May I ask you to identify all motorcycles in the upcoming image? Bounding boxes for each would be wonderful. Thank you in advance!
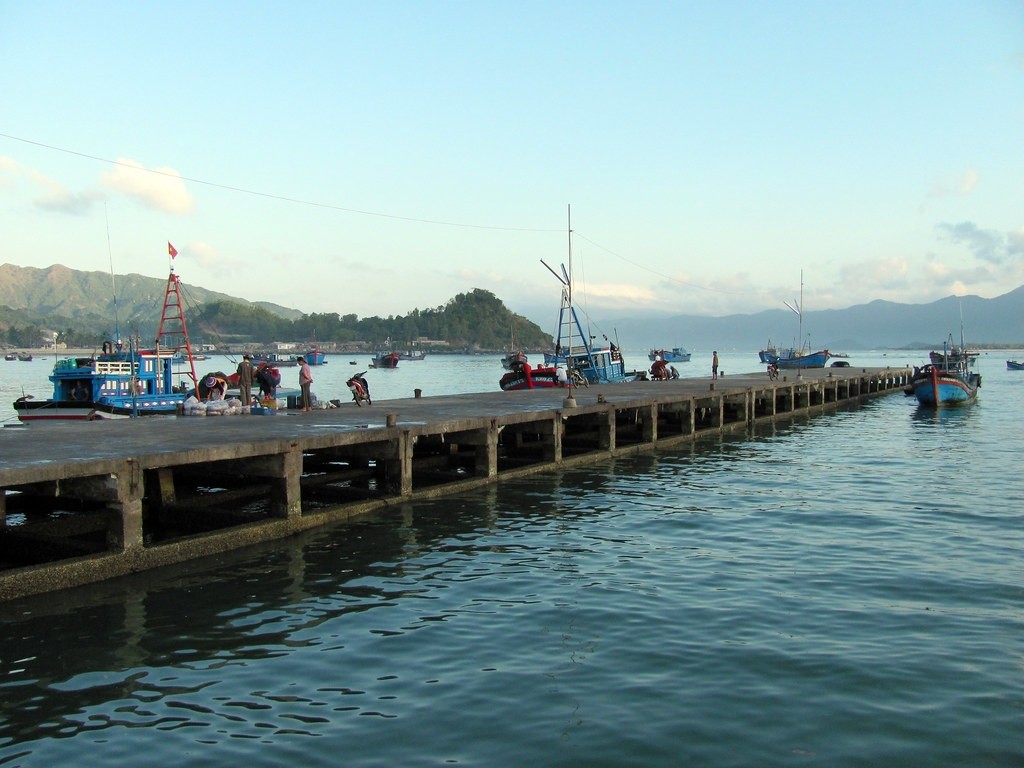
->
[346,371,372,408]
[567,362,590,388]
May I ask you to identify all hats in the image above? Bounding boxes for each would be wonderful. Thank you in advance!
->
[206,377,216,387]
[243,355,249,360]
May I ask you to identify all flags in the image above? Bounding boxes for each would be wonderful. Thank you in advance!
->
[169,243,178,259]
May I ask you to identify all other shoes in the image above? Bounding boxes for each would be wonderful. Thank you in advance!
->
[299,407,311,412]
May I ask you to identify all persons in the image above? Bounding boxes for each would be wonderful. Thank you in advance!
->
[553,367,567,387]
[712,351,718,380]
[296,357,313,412]
[651,351,679,381]
[237,355,254,406]
[253,369,279,397]
[205,377,228,401]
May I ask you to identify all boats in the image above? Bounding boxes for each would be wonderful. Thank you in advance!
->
[396,346,428,362]
[223,387,318,410]
[827,352,851,359]
[563,250,648,386]
[902,300,983,408]
[368,352,401,370]
[542,345,572,363]
[648,344,692,362]
[499,354,568,391]
[242,329,328,367]
[831,361,851,367]
[6,353,32,361]
[13,218,196,424]
[1006,360,1024,370]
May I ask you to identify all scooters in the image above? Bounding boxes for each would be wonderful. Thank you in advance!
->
[767,357,781,380]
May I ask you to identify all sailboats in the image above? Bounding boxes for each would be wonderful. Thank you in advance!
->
[500,324,532,372]
[760,267,830,368]
